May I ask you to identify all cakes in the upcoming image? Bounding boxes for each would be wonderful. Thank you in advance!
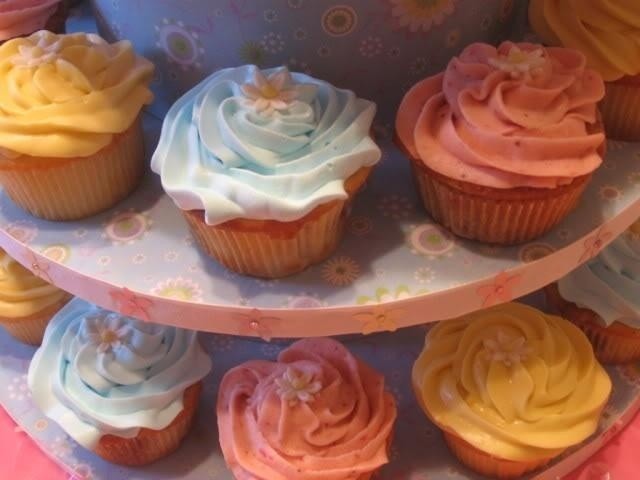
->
[216,336,398,479]
[527,0,640,143]
[149,64,382,278]
[411,300,613,479]
[544,219,640,365]
[0,250,75,347]
[0,1,71,45]
[0,30,156,222]
[28,297,214,466]
[392,39,608,245]
